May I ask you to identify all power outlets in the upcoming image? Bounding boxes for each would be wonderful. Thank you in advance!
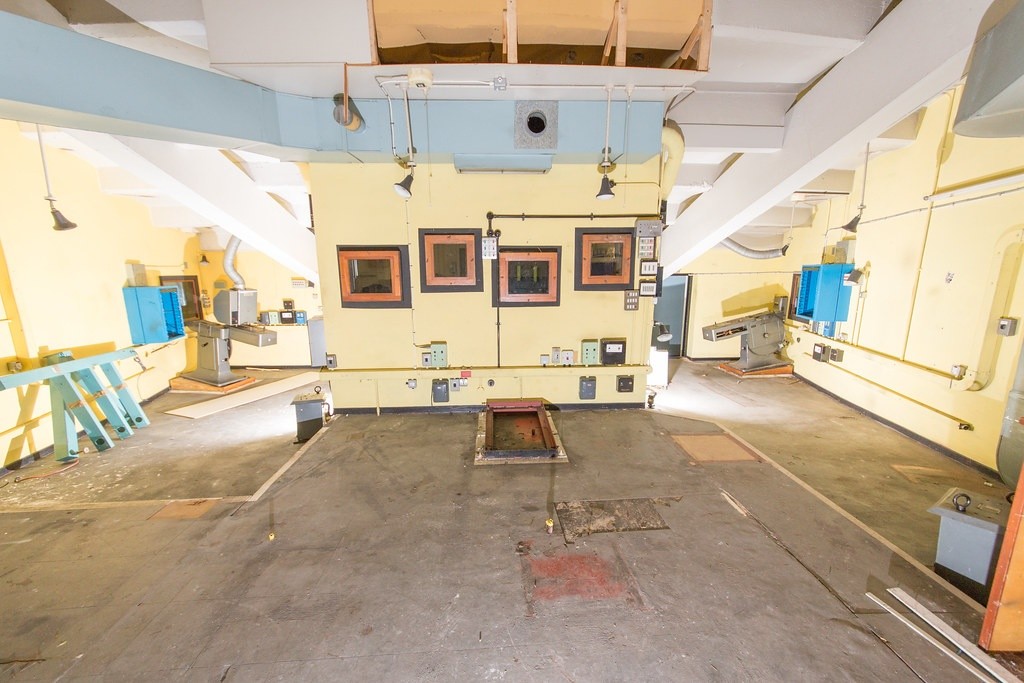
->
[482,237,497,259]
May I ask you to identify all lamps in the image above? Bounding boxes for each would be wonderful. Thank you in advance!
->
[193,253,209,266]
[654,321,673,342]
[597,85,617,199]
[35,123,77,231]
[394,87,416,198]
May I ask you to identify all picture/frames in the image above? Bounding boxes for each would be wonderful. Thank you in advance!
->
[418,228,484,293]
[159,275,204,321]
[574,227,637,292]
[336,244,412,309]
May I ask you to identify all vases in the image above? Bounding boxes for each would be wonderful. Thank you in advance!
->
[491,245,562,307]
